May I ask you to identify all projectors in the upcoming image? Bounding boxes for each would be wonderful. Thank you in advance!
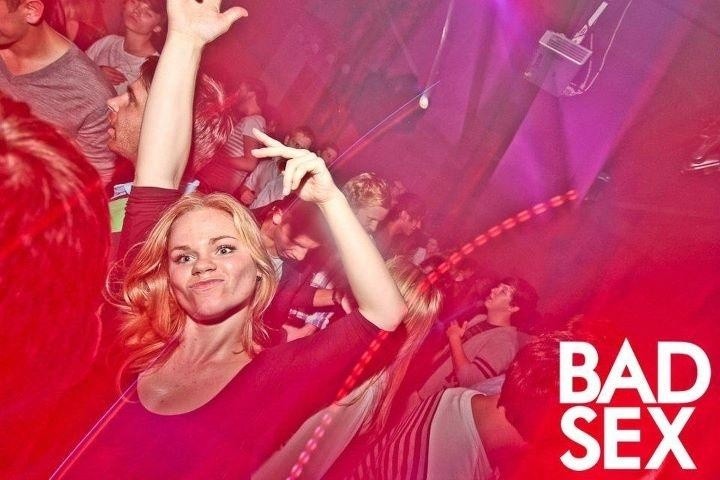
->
[521,29,594,98]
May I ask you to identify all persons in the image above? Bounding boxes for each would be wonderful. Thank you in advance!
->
[2,0,606,479]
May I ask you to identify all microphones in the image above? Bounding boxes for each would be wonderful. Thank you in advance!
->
[585,170,611,202]
